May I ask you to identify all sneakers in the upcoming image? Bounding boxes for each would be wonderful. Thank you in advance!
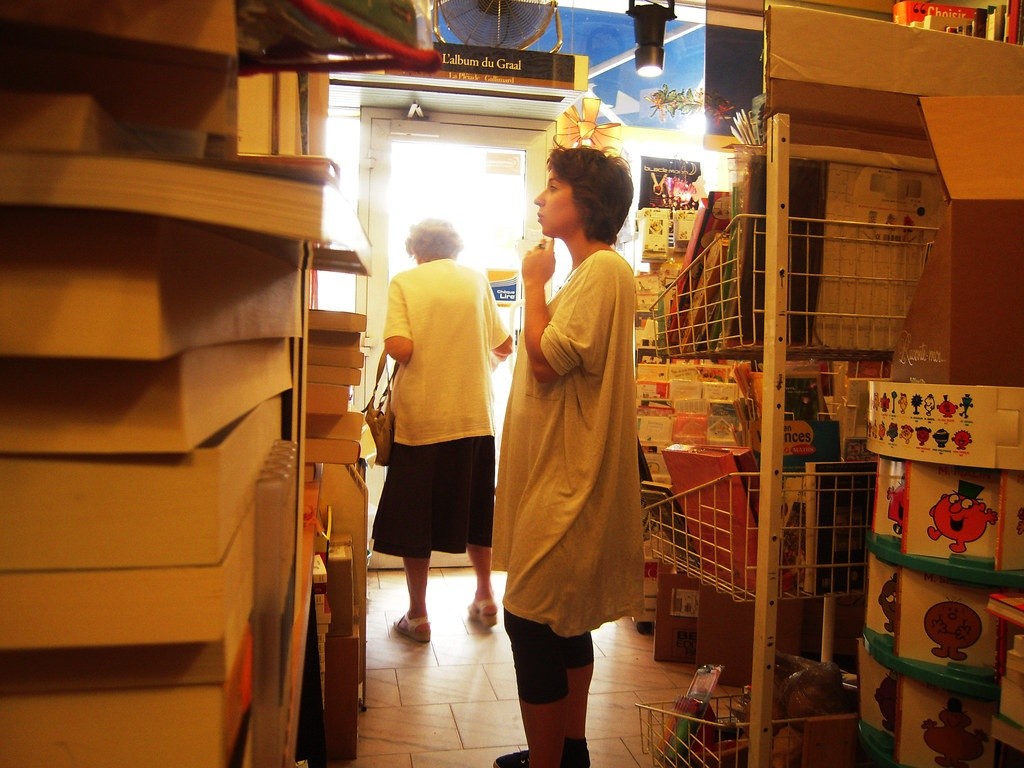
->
[493,750,590,768]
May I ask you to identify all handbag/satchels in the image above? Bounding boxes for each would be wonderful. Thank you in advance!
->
[364,349,401,465]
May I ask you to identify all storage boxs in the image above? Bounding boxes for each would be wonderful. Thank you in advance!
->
[313,532,362,761]
[651,573,803,688]
[1000,635,1024,728]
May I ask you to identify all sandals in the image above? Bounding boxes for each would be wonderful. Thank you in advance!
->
[394,617,431,644]
[469,595,498,626]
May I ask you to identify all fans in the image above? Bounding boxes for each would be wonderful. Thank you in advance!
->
[431,0,564,55]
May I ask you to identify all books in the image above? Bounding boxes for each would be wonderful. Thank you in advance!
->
[633,190,891,594]
[853,452,1024,768]
[0,0,373,768]
[892,0,1024,46]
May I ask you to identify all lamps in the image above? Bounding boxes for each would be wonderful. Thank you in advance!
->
[626,0,678,78]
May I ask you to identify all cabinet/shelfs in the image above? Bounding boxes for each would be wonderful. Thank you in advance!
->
[635,112,945,768]
[856,380,1024,768]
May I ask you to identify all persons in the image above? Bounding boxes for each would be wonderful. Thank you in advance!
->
[373,217,513,643]
[489,134,646,768]
